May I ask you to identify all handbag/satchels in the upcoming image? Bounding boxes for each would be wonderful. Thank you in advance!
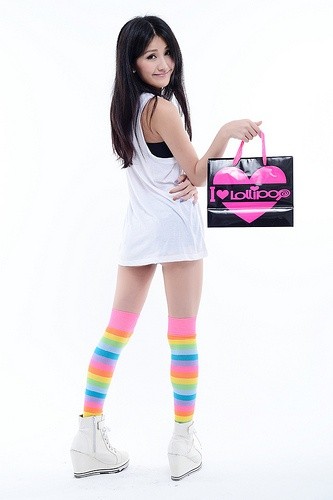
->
[207,130,294,231]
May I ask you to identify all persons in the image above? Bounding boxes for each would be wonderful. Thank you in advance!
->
[72,14,264,479]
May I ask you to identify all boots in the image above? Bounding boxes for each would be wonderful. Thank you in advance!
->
[168,419,203,480]
[68,415,127,478]
